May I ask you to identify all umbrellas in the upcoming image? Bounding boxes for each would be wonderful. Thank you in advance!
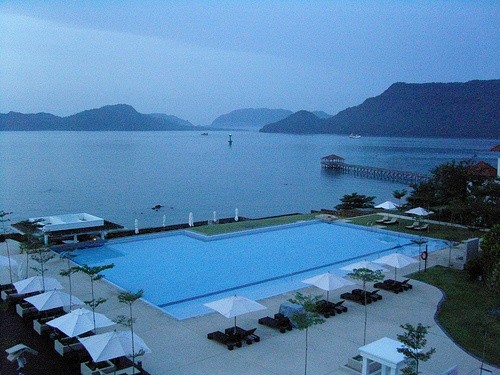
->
[340,258,390,291]
[301,271,354,304]
[12,275,64,294]
[78,329,149,363]
[374,200,402,220]
[45,307,117,338]
[23,287,86,311]
[203,294,267,333]
[371,251,421,282]
[404,206,434,222]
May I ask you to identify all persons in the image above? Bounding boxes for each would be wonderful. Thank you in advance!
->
[16,352,27,375]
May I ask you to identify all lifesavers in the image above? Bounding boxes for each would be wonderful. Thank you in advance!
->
[420,251,428,260]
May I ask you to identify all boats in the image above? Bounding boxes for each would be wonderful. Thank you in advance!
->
[349,134,360,139]
[201,133,208,135]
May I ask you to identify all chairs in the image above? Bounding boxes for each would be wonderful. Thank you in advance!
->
[351,288,383,301]
[224,325,261,346]
[414,223,428,232]
[342,291,372,305]
[405,221,419,230]
[375,216,389,225]
[315,299,347,314]
[311,302,335,318]
[205,330,242,351]
[384,217,398,226]
[257,315,293,332]
[372,282,404,294]
[383,278,413,291]
